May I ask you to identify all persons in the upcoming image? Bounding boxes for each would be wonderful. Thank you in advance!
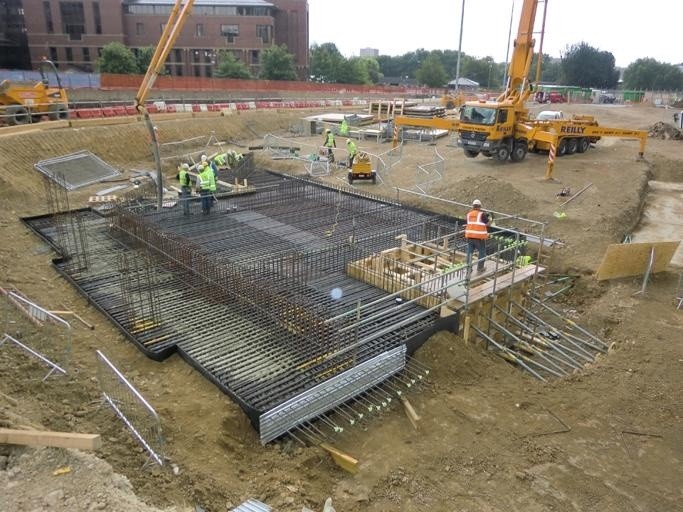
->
[345,139,356,168]
[464,200,492,275]
[174,150,243,214]
[322,129,336,162]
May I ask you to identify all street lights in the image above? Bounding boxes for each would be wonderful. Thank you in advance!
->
[487,60,493,89]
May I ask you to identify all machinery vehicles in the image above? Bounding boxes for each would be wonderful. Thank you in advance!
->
[0,60,69,126]
[386,1,649,182]
[440,90,477,110]
[599,93,616,103]
[534,91,567,104]
[347,152,376,187]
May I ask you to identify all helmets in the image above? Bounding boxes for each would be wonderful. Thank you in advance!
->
[472,199,482,206]
[183,155,208,174]
